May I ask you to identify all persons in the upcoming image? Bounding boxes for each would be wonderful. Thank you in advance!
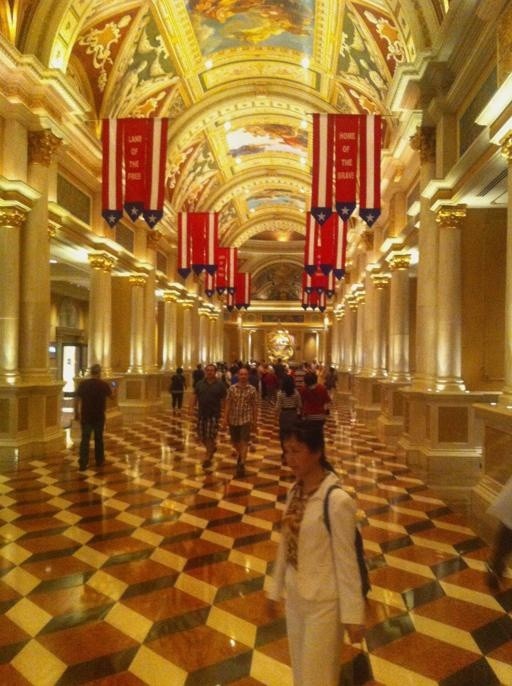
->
[188,356,339,473]
[485,472,512,600]
[268,416,370,684]
[169,367,188,414]
[74,364,118,470]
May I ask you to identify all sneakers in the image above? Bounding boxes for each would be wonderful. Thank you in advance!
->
[202,447,217,467]
[80,460,105,470]
[236,455,245,477]
